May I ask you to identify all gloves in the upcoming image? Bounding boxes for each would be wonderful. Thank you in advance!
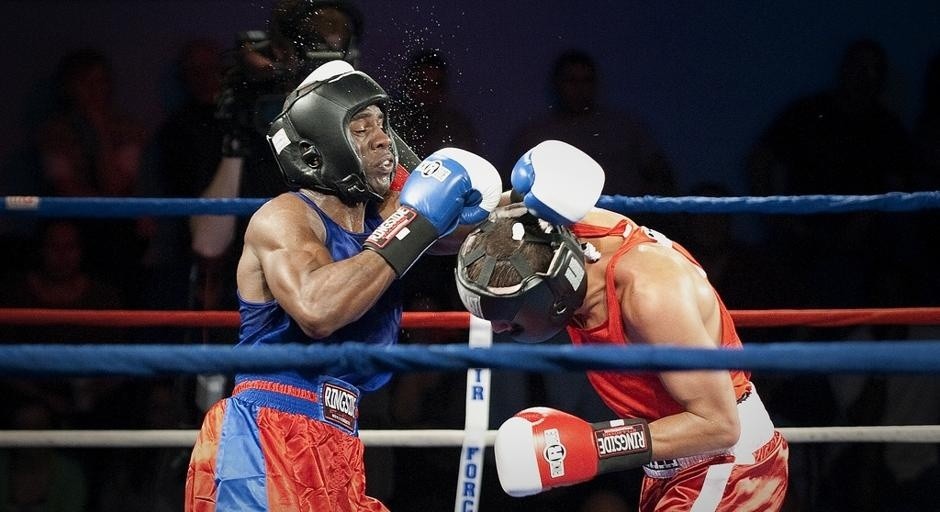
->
[216,71,255,158]
[511,140,606,225]
[396,147,503,237]
[494,405,652,498]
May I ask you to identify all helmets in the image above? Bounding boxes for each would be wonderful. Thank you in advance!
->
[453,215,588,344]
[267,70,398,203]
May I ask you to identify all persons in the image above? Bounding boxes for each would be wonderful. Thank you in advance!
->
[517,35,940,511]
[374,131,788,510]
[360,50,641,510]
[2,1,365,510]
[184,61,605,510]
